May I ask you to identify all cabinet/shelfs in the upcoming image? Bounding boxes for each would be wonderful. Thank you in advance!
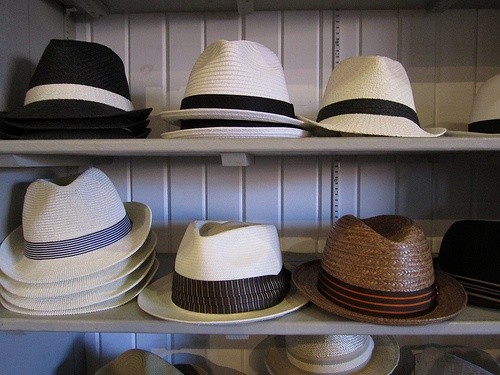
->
[0,138,500,341]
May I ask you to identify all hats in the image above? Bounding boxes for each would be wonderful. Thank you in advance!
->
[0,38,500,375]
